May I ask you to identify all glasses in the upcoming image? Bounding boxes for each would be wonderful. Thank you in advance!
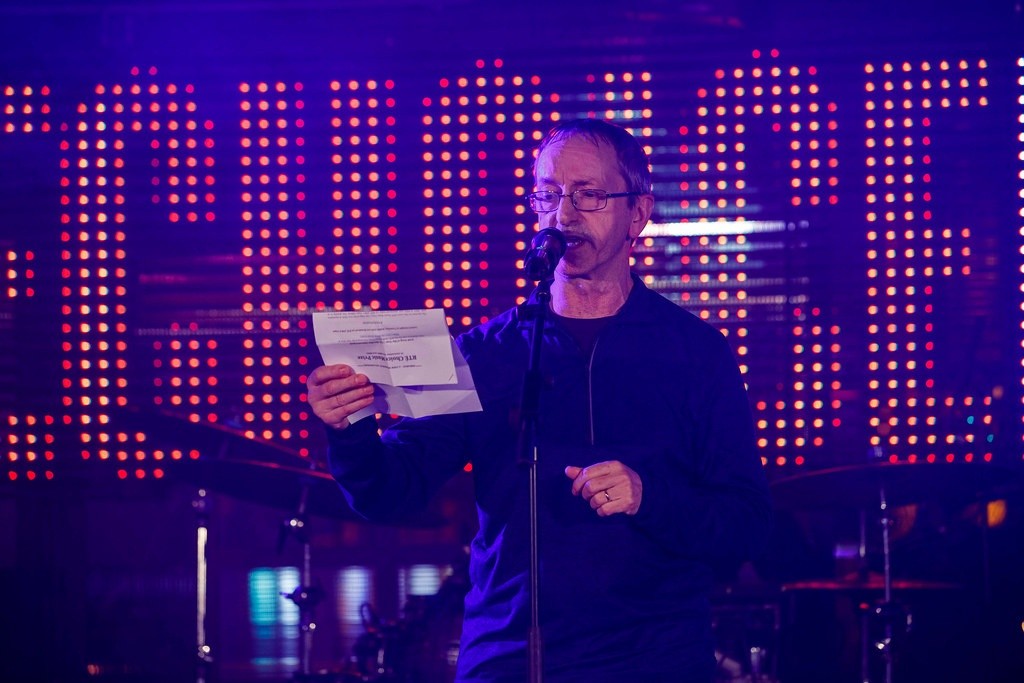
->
[524,186,650,214]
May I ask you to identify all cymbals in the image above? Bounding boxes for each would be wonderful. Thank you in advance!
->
[780,569,960,597]
[179,455,469,532]
[768,455,973,513]
[110,404,314,468]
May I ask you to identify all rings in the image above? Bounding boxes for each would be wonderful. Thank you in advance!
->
[604,490,610,502]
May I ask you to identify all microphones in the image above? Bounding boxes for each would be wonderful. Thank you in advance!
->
[524,225,568,281]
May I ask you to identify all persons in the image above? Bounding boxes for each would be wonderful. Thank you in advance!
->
[306,116,762,682]
